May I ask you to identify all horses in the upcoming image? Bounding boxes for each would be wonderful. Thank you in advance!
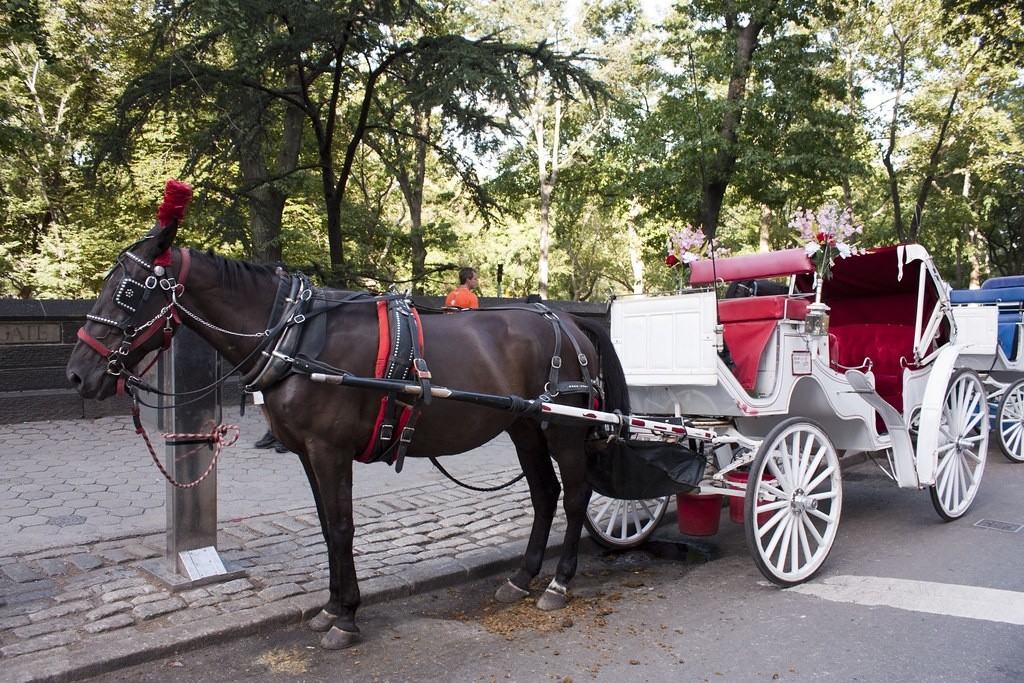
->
[66,224,629,651]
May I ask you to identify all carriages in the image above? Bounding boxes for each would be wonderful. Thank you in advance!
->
[948,275,1023,465]
[65,177,992,650]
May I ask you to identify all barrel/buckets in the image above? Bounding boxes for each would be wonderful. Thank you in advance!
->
[727,472,775,524]
[677,494,722,535]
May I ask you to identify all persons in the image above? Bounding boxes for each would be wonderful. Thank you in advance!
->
[444,265,479,311]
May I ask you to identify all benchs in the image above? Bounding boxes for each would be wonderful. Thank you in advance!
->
[803,291,944,434]
[680,247,817,324]
[951,277,1024,359]
